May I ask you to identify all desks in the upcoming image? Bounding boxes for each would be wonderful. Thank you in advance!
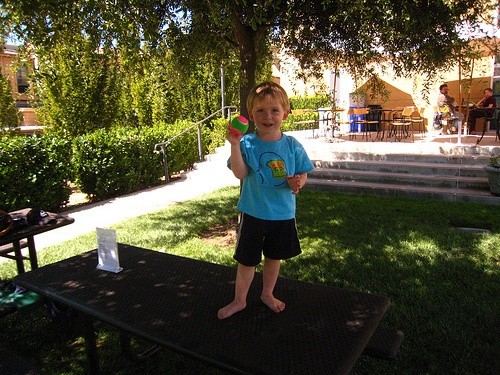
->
[13,241,392,375]
[318,108,403,142]
[454,105,476,134]
[0,206,75,275]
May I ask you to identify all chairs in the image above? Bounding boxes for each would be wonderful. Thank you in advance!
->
[327,106,427,142]
[435,95,500,144]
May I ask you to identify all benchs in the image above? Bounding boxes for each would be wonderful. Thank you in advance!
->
[0,282,48,318]
[362,325,405,362]
[0,240,30,261]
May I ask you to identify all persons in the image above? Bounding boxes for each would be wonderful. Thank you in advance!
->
[437,84,464,131]
[468,88,496,134]
[218,81,315,319]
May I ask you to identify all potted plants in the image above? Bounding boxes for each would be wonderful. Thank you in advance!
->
[484,153,500,195]
